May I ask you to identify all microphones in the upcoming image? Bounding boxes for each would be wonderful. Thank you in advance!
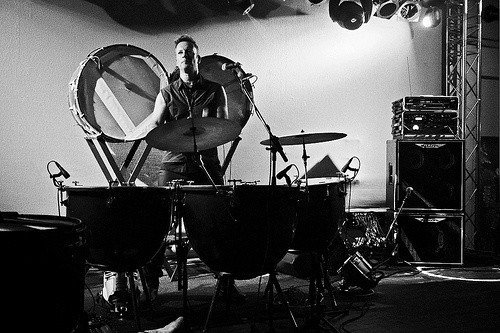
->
[221,62,241,71]
[277,164,293,180]
[341,158,353,173]
[55,162,70,179]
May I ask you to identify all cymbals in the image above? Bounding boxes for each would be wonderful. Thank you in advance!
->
[145,117,242,152]
[260,132,347,145]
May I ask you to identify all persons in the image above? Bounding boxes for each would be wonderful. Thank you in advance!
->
[132,36,249,301]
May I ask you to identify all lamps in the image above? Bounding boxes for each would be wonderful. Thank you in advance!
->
[307,0,441,30]
[226,0,254,15]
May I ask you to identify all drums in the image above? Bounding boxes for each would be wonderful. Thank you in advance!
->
[0,211,86,333]
[181,184,302,280]
[293,179,347,252]
[66,184,175,273]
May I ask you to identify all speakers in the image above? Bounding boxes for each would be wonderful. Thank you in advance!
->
[384,140,466,267]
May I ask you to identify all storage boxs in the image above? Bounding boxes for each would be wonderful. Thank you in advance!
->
[391,95,459,139]
